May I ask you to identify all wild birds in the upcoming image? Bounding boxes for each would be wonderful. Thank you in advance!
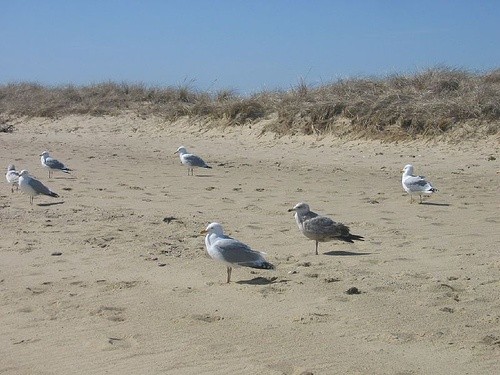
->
[19,170,60,206]
[6,164,21,192]
[40,151,72,178]
[200,222,276,283]
[288,203,365,255]
[174,146,212,176]
[400,165,439,203]
